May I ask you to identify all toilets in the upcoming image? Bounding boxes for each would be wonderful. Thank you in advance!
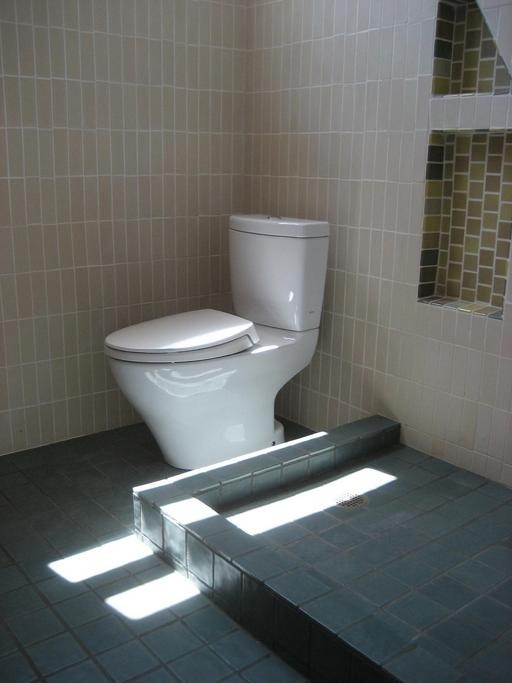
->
[102,212,335,473]
